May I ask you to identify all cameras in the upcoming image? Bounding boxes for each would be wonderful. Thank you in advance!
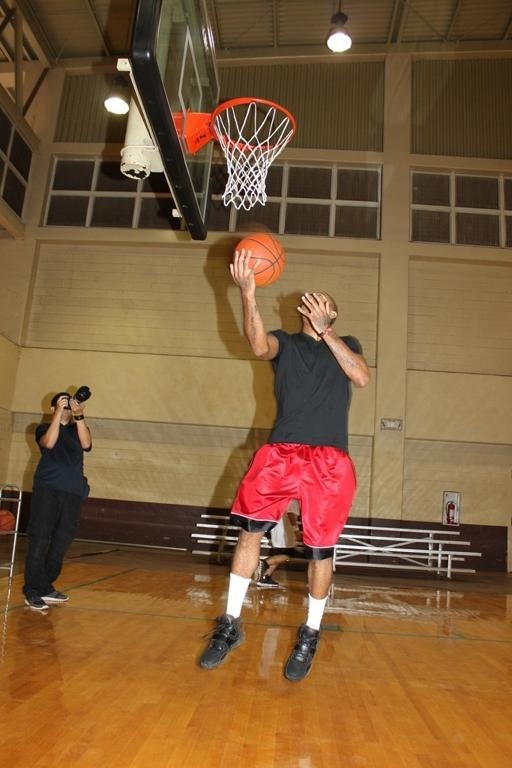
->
[61,386,91,409]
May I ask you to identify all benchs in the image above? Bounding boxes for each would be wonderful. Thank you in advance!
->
[188,511,481,581]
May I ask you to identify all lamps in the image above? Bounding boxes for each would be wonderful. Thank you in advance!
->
[325,0,353,54]
[100,78,130,118]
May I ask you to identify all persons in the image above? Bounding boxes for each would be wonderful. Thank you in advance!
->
[21,392,92,609]
[199,247,370,682]
[253,496,300,587]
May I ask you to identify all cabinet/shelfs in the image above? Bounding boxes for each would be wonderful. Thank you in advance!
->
[0,482,22,578]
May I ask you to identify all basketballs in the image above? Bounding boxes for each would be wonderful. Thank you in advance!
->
[0,510,15,531]
[233,233,286,288]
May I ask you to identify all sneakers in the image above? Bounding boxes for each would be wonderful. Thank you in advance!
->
[200,613,245,668]
[41,591,70,602]
[284,623,321,681]
[257,575,278,587]
[23,596,48,610]
[255,559,269,581]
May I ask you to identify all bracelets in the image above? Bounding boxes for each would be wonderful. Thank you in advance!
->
[293,525,299,531]
[74,415,84,420]
[319,326,332,337]
